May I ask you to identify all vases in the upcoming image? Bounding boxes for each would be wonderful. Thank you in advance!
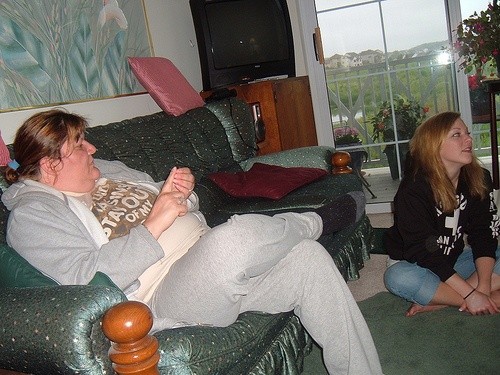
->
[491,54,500,78]
[385,148,410,181]
[336,139,363,174]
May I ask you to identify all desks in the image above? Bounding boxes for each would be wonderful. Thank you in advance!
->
[481,78,500,191]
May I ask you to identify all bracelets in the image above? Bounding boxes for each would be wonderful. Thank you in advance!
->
[464,288,475,300]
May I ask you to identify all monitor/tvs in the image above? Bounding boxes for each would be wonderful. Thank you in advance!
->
[189,0,295,91]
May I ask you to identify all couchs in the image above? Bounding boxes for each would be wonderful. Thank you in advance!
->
[0,92,376,375]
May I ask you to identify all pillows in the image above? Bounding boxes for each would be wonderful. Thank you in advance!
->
[128,57,206,117]
[206,162,328,199]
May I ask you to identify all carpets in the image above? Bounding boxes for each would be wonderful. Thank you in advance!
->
[301,292,500,375]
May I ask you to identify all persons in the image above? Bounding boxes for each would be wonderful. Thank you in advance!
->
[0,108,385,375]
[384,111,500,316]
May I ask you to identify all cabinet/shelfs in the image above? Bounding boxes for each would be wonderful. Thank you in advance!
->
[198,75,317,156]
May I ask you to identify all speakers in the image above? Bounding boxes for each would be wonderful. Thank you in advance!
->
[247,102,265,143]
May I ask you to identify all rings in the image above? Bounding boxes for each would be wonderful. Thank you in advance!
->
[189,187,194,191]
[178,199,181,204]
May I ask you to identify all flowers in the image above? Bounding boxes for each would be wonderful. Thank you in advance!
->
[363,94,430,151]
[447,0,500,91]
[333,121,361,144]
